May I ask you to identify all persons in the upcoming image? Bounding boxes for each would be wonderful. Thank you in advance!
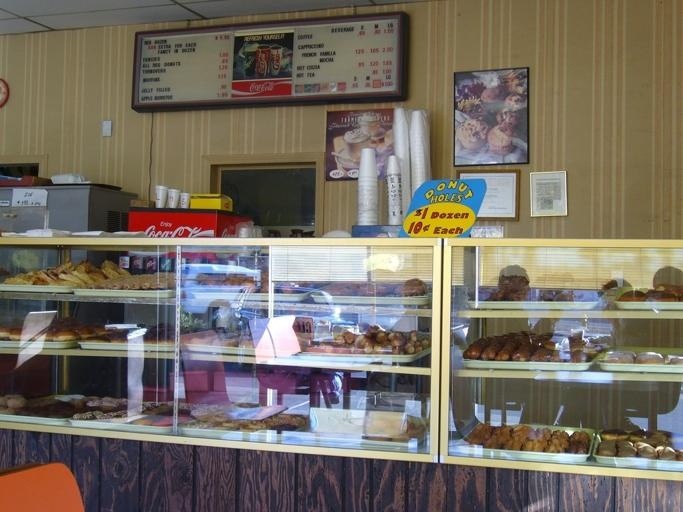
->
[364,262,683,432]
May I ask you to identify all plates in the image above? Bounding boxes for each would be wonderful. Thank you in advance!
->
[335,149,377,169]
[355,127,386,141]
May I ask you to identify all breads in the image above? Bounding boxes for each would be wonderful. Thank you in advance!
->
[457,86,527,154]
[463,331,683,366]
[180,405,429,441]
[2,316,175,343]
[466,423,683,461]
[4,261,186,291]
[196,273,426,297]
[469,285,683,302]
[213,321,431,355]
[2,393,194,425]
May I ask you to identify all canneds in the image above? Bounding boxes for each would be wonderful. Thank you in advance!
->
[269,47,281,76]
[256,45,269,76]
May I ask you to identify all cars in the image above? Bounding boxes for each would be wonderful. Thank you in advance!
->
[170,264,342,340]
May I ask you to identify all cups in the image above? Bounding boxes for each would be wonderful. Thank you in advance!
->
[392,106,433,210]
[357,148,378,226]
[357,116,380,134]
[154,186,190,209]
[385,154,404,226]
[344,130,371,159]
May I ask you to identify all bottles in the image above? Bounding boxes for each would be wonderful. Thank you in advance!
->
[244,40,260,76]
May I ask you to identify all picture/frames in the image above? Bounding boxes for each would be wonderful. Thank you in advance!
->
[130,11,408,113]
[454,169,567,222]
[451,66,529,166]
